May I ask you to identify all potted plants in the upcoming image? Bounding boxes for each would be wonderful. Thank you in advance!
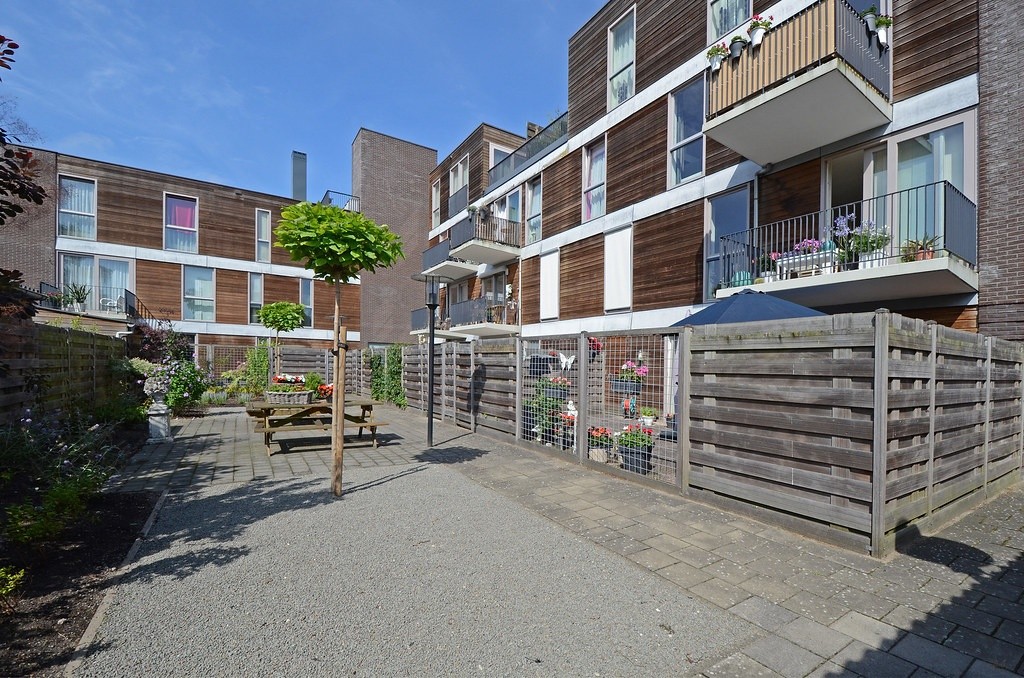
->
[861,6,892,46]
[62,284,92,311]
[464,205,492,220]
[902,231,943,260]
[642,407,657,427]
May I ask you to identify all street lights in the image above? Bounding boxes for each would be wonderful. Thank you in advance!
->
[411,272,461,447]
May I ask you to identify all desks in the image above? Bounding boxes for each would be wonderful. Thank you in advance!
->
[246,402,384,455]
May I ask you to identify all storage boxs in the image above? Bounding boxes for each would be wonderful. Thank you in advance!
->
[266,381,315,404]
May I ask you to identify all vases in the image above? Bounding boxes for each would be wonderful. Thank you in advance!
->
[51,301,60,310]
[756,250,887,286]
[538,381,652,475]
[695,28,772,71]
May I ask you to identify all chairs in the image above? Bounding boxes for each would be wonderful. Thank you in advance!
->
[100,295,124,314]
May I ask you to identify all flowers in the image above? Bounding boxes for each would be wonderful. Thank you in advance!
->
[46,291,61,300]
[262,371,337,401]
[709,13,772,52]
[759,213,886,261]
[544,340,659,448]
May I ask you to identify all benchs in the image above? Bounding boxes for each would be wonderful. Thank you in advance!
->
[250,409,391,459]
